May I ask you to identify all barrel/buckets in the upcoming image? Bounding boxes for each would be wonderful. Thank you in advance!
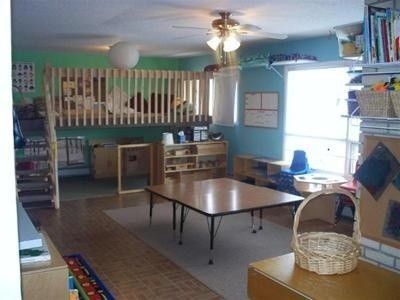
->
[161,132,174,145]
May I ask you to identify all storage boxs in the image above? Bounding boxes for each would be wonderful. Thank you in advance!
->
[333,20,362,61]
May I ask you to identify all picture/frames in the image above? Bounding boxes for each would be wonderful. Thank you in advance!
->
[12,61,35,93]
[244,90,279,129]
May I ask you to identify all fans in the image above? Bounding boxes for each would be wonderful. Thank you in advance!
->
[171,10,289,40]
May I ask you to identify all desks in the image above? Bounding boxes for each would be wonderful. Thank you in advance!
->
[294,172,349,224]
[247,252,400,300]
[145,177,305,266]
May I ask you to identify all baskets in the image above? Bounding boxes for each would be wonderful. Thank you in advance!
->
[290,188,361,275]
[355,90,400,118]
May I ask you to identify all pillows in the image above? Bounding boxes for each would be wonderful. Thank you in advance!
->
[124,92,174,113]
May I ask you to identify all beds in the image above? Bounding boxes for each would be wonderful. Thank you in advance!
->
[53,95,212,125]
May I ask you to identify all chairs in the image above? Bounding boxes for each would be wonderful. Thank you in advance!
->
[278,150,311,192]
[333,160,360,226]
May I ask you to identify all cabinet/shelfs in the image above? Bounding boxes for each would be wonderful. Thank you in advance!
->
[151,140,229,206]
[355,1,400,136]
[90,148,152,181]
[233,154,290,210]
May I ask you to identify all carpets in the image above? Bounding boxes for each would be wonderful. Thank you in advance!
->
[102,202,301,300]
[64,253,113,300]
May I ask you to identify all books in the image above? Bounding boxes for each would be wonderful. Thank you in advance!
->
[364,5,400,65]
